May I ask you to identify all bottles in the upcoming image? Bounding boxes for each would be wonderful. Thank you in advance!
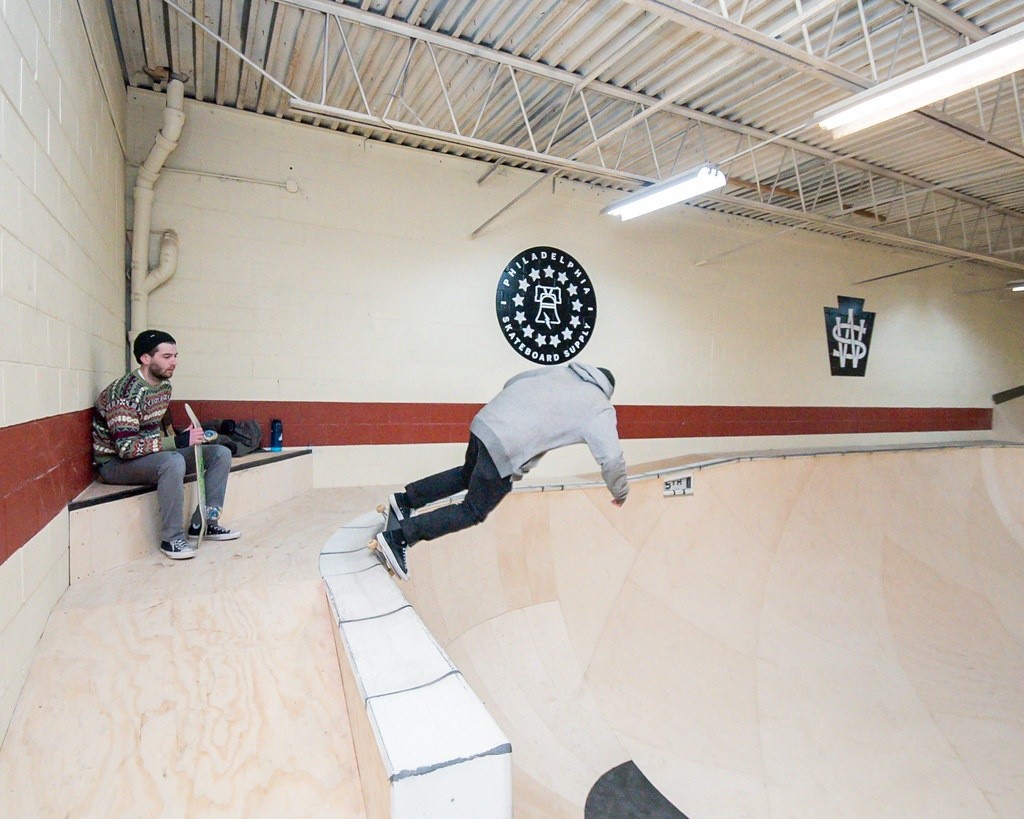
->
[270,418,282,452]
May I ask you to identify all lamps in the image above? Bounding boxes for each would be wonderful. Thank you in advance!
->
[1006,279,1024,291]
[812,21,1024,141]
[599,162,726,222]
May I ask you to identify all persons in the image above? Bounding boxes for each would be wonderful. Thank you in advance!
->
[93,330,240,559]
[376,362,631,581]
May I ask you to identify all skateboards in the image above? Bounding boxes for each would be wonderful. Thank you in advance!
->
[367,502,410,581]
[184,402,222,551]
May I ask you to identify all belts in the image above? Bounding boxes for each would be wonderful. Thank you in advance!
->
[99,460,111,468]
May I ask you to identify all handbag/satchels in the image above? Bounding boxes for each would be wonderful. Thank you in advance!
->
[201,419,261,457]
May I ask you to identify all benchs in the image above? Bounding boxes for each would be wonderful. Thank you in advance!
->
[69,449,313,584]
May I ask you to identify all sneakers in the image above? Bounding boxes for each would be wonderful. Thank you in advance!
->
[376,529,409,579]
[188,522,241,541]
[159,538,196,559]
[390,492,412,522]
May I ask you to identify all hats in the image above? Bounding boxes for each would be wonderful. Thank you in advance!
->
[133,330,176,364]
[598,367,615,388]
